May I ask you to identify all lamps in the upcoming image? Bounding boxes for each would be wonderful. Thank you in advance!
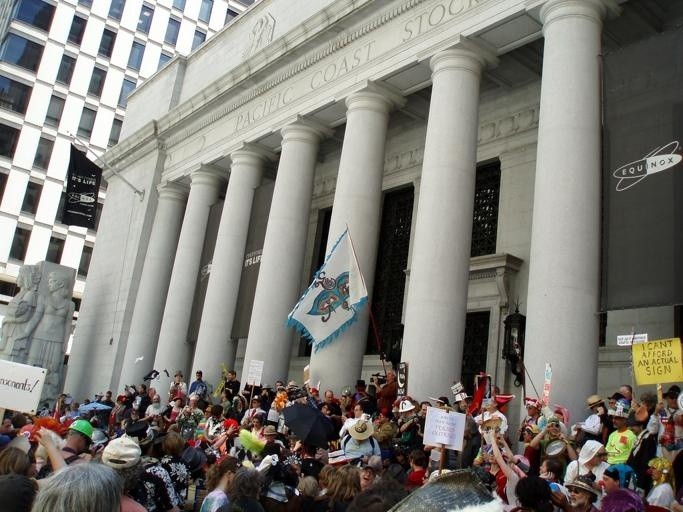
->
[502,296,526,388]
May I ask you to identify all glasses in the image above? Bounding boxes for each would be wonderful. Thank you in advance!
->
[595,453,607,457]
[568,488,580,493]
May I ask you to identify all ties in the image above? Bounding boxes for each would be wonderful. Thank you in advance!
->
[251,409,256,417]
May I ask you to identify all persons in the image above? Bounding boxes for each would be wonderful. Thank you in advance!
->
[13,271,76,385]
[0,263,40,365]
[1,368,683,510]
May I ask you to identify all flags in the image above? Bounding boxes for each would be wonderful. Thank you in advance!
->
[62,143,103,229]
[286,229,369,354]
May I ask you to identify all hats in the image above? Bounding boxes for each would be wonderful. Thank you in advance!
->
[663,385,680,398]
[8,420,158,469]
[252,395,262,400]
[182,448,206,472]
[453,393,473,404]
[260,384,273,389]
[587,392,630,418]
[263,425,277,434]
[565,470,598,496]
[355,380,366,387]
[224,419,238,430]
[525,398,542,410]
[398,400,415,412]
[363,465,377,476]
[349,420,374,440]
[604,463,637,490]
